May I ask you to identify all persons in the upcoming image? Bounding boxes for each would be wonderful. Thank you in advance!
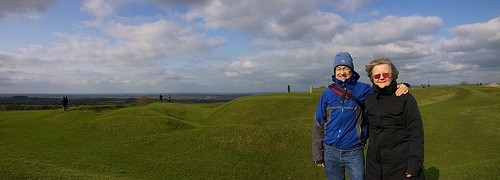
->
[160,94,163,102]
[312,52,412,180]
[62,96,69,112]
[362,58,427,180]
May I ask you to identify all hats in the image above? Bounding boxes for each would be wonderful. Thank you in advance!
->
[333,52,354,72]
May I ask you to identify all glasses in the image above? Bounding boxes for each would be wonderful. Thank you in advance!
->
[371,73,393,79]
